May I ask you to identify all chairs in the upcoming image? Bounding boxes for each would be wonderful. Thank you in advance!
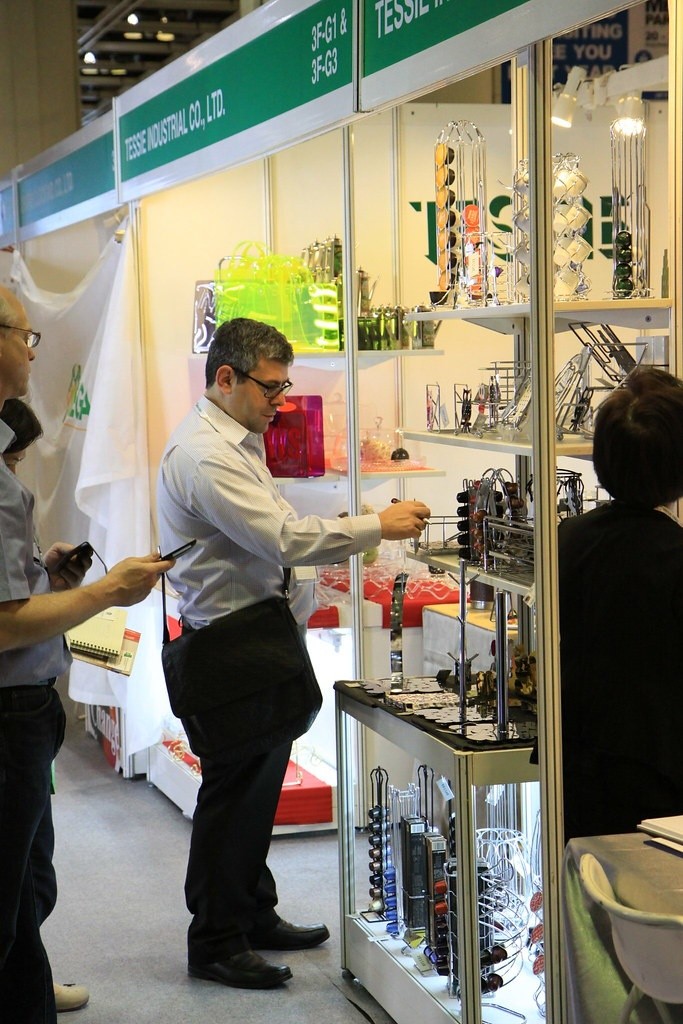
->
[580,853,683,1024]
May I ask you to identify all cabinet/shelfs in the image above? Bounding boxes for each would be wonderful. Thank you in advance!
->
[402,300,672,677]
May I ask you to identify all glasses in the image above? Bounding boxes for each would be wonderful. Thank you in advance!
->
[230,364,293,398]
[0,324,41,348]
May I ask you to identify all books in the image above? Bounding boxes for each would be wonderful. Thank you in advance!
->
[62,607,141,676]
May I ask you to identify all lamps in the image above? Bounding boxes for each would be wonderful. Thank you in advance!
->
[610,87,645,135]
[551,67,585,127]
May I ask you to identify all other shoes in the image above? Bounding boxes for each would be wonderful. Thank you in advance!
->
[52,981,88,1010]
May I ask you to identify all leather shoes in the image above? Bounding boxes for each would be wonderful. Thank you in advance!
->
[187,950,292,989]
[249,913,330,950]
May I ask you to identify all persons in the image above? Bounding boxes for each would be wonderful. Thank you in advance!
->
[0,281,176,1024]
[157,315,431,989]
[529,369,682,844]
[1,397,89,1011]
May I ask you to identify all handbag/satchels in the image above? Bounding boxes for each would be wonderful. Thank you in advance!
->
[161,596,324,762]
